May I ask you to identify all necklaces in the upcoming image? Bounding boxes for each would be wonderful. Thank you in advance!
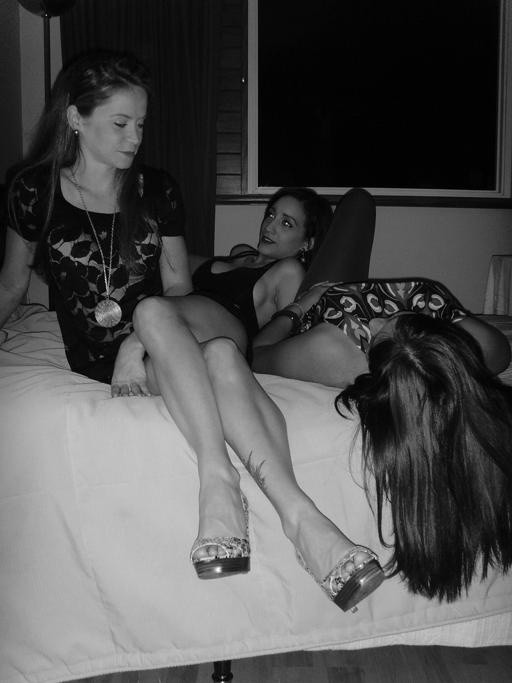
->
[61,169,124,326]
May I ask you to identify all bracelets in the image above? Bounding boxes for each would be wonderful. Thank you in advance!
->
[284,302,304,319]
[272,309,302,334]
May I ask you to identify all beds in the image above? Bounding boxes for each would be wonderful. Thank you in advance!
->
[1,295,511,680]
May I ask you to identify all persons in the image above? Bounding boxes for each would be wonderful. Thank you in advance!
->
[108,182,332,398]
[0,63,385,614]
[244,276,512,598]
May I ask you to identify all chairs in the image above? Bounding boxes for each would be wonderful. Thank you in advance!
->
[294,188,376,309]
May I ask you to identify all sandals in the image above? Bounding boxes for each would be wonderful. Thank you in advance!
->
[191,490,252,579]
[294,542,387,612]
